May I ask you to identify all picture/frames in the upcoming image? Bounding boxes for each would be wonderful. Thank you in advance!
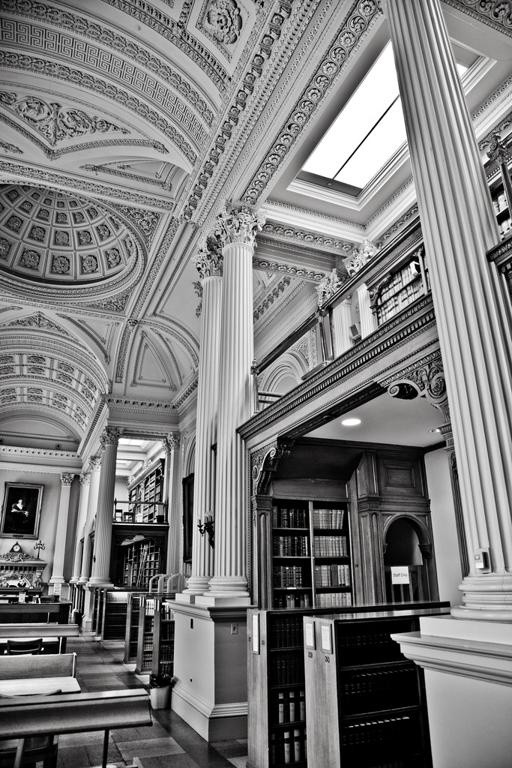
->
[0,481,45,541]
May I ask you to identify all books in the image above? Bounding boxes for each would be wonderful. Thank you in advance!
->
[269,500,356,688]
[127,469,164,525]
[105,599,174,679]
[123,553,159,587]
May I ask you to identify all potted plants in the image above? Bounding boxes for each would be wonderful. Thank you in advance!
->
[146,674,174,711]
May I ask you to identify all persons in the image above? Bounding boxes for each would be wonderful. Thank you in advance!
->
[13,498,28,511]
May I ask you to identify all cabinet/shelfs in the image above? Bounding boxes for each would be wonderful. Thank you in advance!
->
[104,440,173,682]
[253,474,349,611]
[243,608,380,767]
[302,607,453,767]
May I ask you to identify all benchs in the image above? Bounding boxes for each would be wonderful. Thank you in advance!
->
[0,587,153,768]
